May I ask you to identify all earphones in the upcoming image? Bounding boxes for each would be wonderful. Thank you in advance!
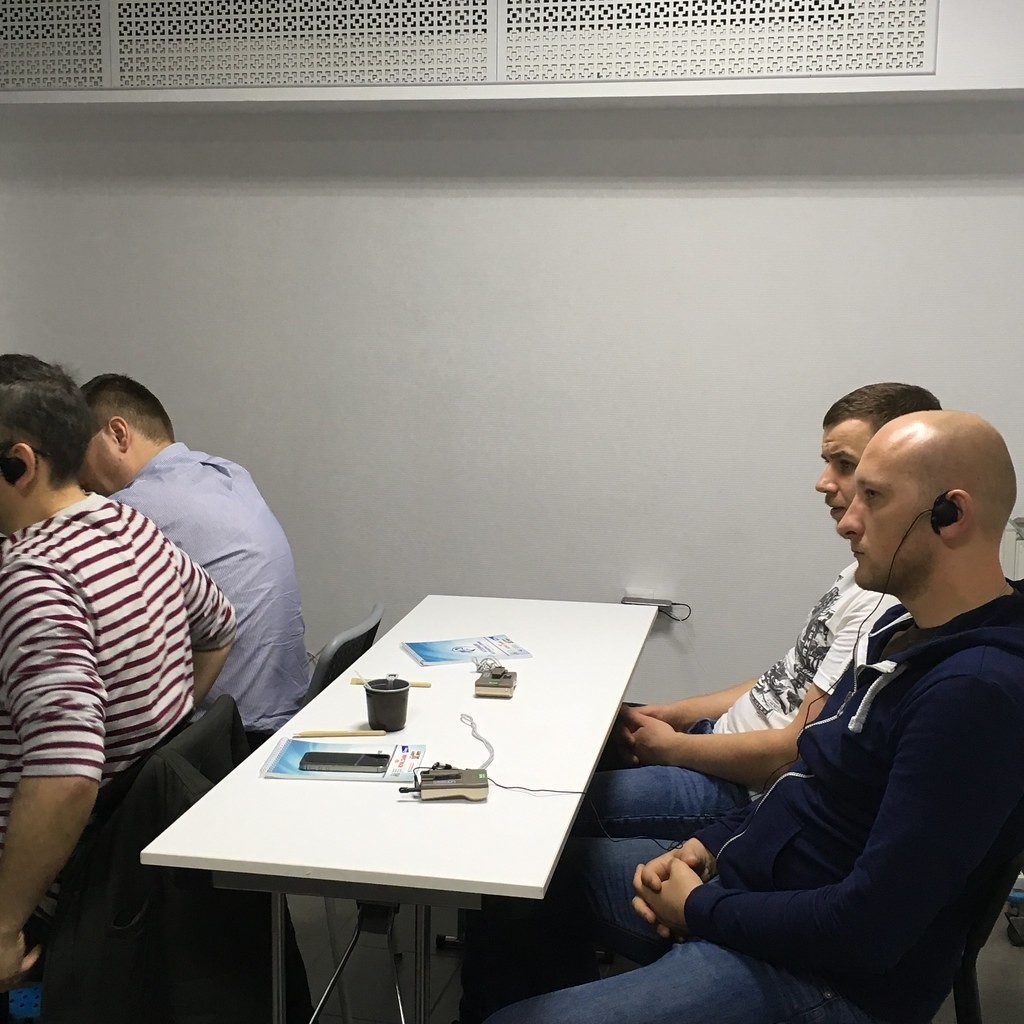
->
[0,456,26,484]
[931,490,960,535]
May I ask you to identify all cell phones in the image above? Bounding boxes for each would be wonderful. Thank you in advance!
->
[300,752,391,772]
[621,597,672,607]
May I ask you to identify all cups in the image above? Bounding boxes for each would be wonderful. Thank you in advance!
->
[365,679,410,731]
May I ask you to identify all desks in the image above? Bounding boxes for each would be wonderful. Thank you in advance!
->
[141,589,660,1024]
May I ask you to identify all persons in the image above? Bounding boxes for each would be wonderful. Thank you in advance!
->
[458,383,1024,1024]
[77,372,311,755]
[0,354,239,993]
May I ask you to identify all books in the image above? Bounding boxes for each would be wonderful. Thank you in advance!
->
[259,738,426,782]
[401,634,532,666]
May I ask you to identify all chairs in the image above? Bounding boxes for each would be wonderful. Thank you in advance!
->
[0,598,386,1024]
[951,797,1023,1023]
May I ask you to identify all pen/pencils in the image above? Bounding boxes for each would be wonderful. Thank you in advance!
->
[351,677,432,687]
[292,730,386,736]
